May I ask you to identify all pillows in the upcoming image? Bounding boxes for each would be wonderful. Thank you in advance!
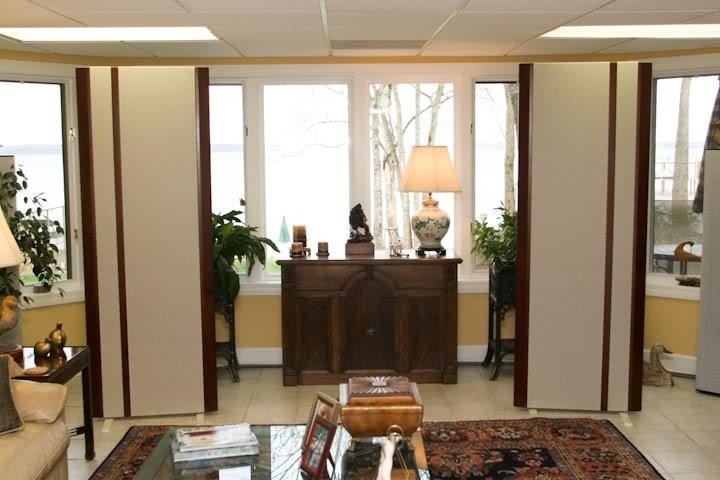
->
[0,354,24,432]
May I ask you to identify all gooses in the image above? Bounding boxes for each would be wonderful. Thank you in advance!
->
[642,342,674,387]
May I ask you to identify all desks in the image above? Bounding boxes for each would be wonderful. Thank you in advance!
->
[652,243,702,275]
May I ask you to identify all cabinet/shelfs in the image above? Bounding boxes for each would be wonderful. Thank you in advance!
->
[276,258,462,389]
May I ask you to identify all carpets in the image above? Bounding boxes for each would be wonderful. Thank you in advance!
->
[85,418,664,480]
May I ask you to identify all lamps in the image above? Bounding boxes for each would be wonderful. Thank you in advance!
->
[396,144,464,257]
[0,202,31,434]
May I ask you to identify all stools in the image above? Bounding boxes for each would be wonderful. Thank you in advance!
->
[334,373,431,479]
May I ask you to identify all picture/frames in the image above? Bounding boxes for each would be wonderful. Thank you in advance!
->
[301,418,337,480]
[301,391,341,452]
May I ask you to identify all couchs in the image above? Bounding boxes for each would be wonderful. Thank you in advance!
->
[0,379,73,480]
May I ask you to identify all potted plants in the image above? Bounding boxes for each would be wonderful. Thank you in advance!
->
[209,211,280,382]
[472,202,518,382]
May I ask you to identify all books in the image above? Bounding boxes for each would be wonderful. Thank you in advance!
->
[169,421,261,462]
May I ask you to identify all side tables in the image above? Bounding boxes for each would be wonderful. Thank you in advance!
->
[11,344,97,464]
